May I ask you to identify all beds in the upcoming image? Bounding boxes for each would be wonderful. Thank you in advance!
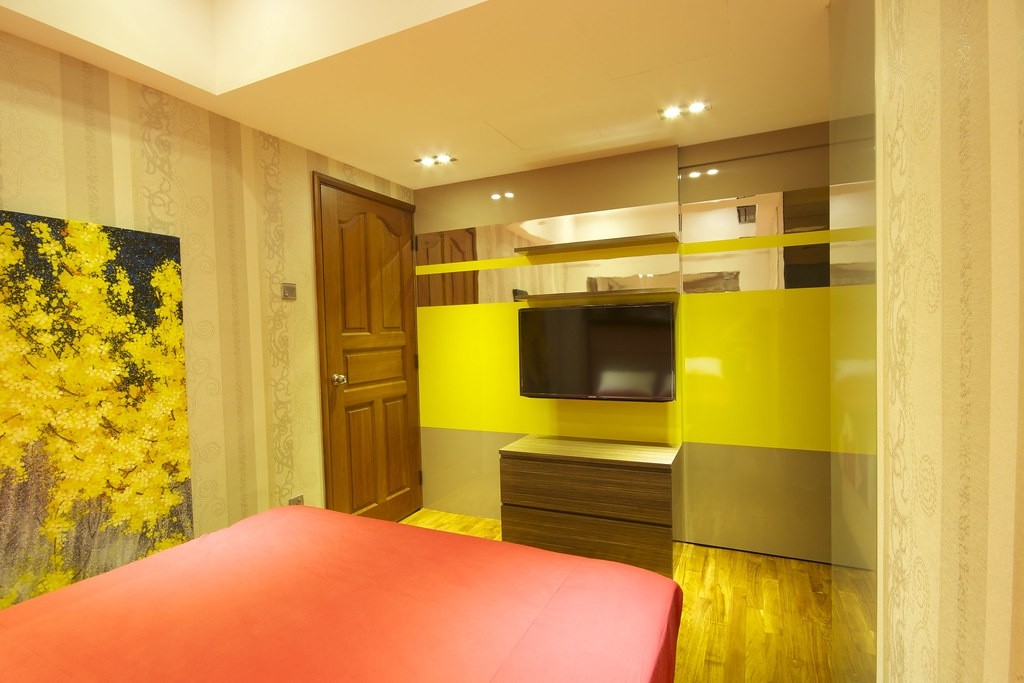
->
[0,505,683,683]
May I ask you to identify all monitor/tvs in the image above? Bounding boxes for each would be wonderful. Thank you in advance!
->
[518,301,675,402]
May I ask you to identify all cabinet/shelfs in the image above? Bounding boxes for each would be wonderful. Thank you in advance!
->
[499,433,684,580]
